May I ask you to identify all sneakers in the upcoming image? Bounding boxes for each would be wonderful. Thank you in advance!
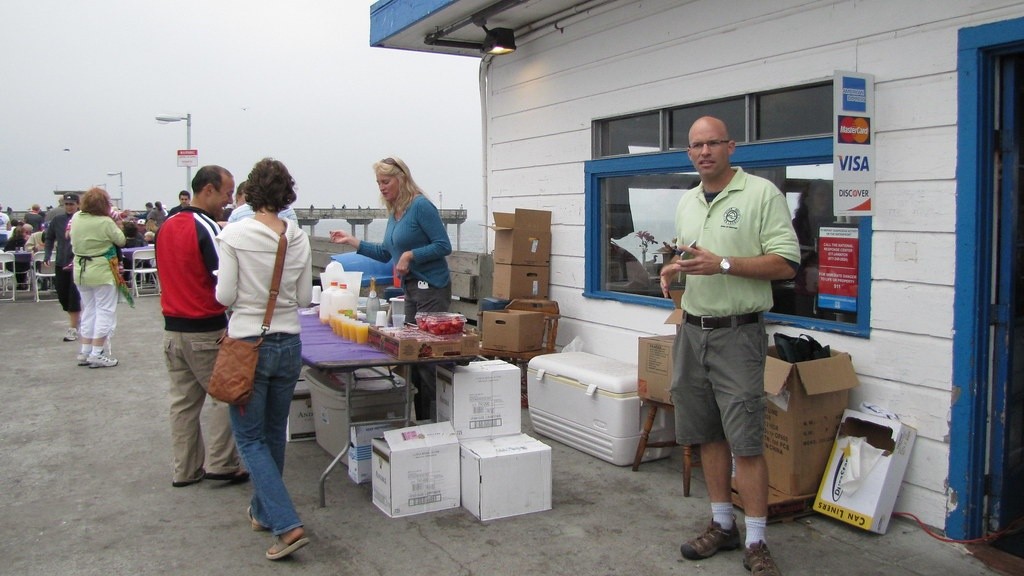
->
[680,519,742,560]
[744,540,782,575]
[87,350,118,368]
[63,328,79,341]
[77,351,92,366]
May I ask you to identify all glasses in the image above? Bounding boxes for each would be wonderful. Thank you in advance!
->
[381,158,406,176]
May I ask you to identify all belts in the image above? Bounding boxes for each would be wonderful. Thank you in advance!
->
[681,311,764,330]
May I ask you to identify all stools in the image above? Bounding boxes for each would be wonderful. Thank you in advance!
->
[633,398,709,497]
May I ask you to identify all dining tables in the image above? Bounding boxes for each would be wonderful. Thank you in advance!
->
[291,311,485,509]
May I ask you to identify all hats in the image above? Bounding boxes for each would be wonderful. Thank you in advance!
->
[63,192,79,204]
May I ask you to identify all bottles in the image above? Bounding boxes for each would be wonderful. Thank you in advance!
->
[15,247,23,254]
[366,277,381,324]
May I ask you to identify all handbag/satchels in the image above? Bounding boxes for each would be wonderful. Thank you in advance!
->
[206,331,263,406]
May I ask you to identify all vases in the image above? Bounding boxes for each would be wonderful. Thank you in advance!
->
[641,249,650,274]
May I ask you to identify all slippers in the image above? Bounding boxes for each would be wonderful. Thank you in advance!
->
[205,467,251,481]
[248,505,268,530]
[172,470,205,486]
[265,536,311,559]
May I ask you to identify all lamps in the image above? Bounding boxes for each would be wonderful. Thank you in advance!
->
[474,16,517,57]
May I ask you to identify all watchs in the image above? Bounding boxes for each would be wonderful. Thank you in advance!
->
[719,255,731,275]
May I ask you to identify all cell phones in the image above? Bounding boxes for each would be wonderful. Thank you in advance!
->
[679,240,696,282]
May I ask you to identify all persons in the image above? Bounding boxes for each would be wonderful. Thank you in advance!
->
[215,178,251,228]
[74,186,127,369]
[215,157,316,561]
[1,176,193,296]
[657,113,806,576]
[227,203,300,231]
[329,155,452,422]
[43,192,81,342]
[152,164,252,490]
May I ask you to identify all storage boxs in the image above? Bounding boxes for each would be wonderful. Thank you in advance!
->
[434,359,521,440]
[721,475,806,519]
[479,307,546,351]
[636,333,699,406]
[482,345,549,407]
[284,368,318,443]
[524,350,679,466]
[452,433,557,522]
[490,251,553,304]
[755,342,859,496]
[345,421,418,487]
[368,324,485,362]
[478,207,554,265]
[303,363,419,463]
[369,418,462,519]
[501,297,562,351]
[664,288,693,335]
[811,408,917,535]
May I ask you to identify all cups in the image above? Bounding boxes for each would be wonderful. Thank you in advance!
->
[328,312,370,344]
[376,311,387,326]
[311,286,322,304]
[392,314,406,327]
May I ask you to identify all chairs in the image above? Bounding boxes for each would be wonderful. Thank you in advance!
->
[0,243,171,303]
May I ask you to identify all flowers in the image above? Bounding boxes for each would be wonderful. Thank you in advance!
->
[631,230,659,253]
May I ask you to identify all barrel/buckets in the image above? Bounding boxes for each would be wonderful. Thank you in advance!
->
[319,282,357,324]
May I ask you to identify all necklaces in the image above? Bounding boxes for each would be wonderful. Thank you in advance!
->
[256,211,267,217]
